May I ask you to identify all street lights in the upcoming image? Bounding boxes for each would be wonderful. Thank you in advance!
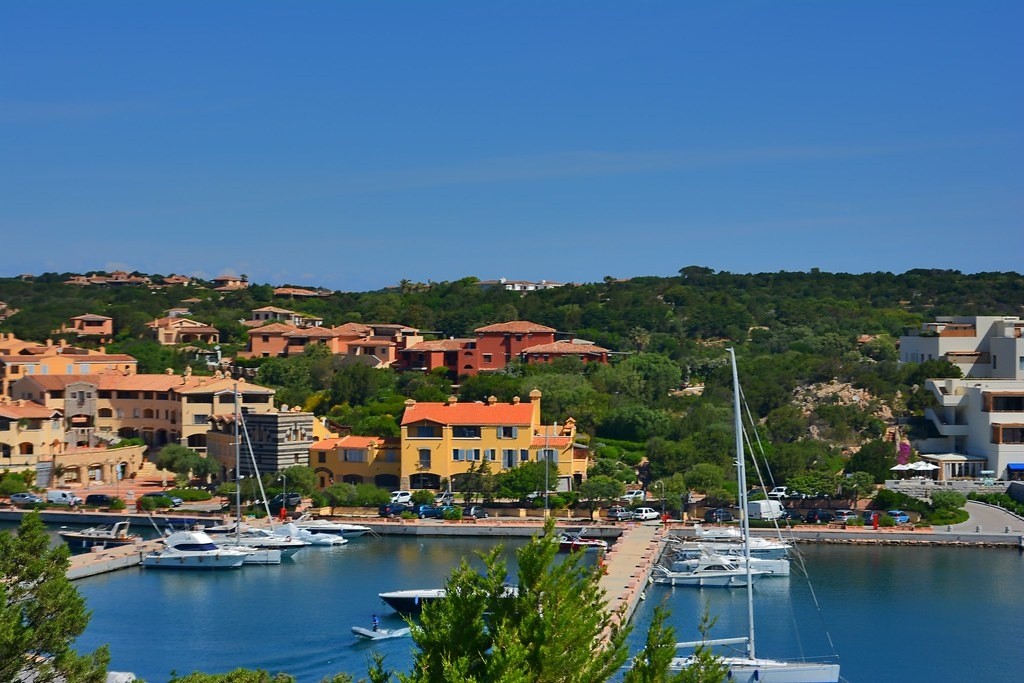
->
[277,474,286,508]
[654,481,665,515]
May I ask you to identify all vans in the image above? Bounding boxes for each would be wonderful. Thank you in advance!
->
[47,490,82,504]
[747,501,785,521]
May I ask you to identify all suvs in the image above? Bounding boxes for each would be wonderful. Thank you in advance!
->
[86,494,116,506]
[143,492,183,508]
[607,508,660,522]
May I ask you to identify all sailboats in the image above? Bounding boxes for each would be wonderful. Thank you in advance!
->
[619,347,841,683]
[532,425,609,553]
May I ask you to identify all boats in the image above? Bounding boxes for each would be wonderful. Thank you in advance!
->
[650,509,794,587]
[352,627,384,638]
[193,383,369,564]
[138,505,257,566]
[378,586,531,613]
[57,516,143,547]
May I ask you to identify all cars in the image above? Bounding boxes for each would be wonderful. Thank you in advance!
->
[782,509,910,526]
[748,486,793,500]
[389,491,413,505]
[433,493,454,502]
[620,490,644,502]
[378,503,489,519]
[10,493,44,504]
[704,509,734,523]
